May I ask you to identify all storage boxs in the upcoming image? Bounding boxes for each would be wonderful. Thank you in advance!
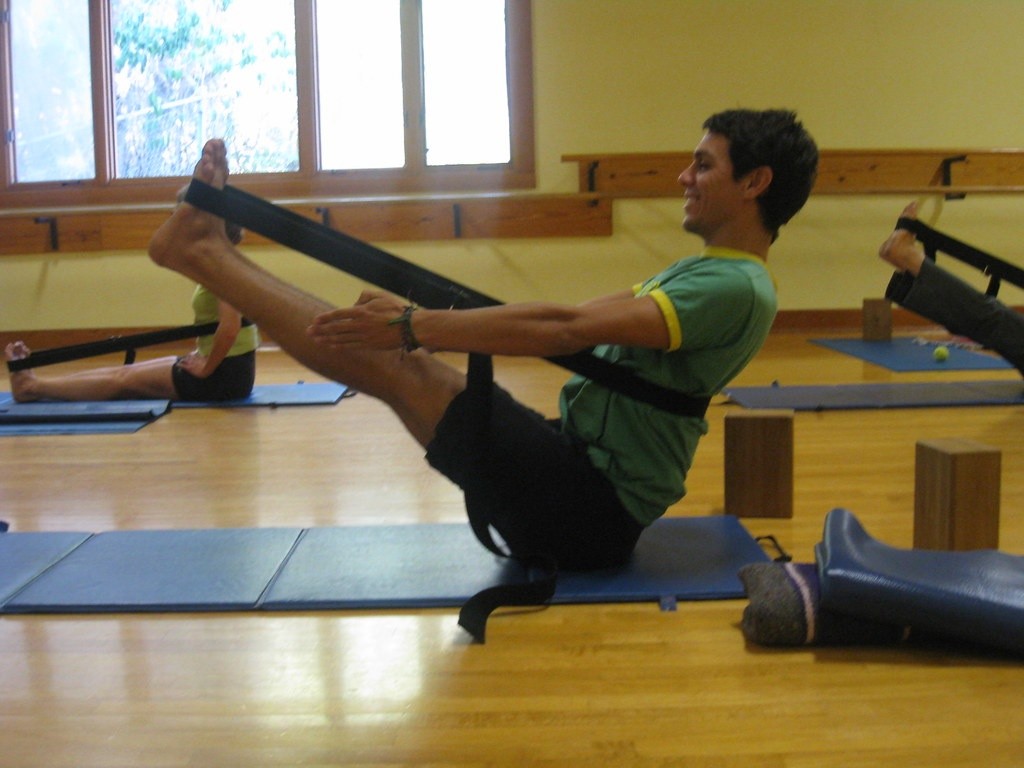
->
[913,438,1002,554]
[725,414,794,520]
[862,295,894,341]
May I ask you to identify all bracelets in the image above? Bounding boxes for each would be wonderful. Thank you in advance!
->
[388,305,423,361]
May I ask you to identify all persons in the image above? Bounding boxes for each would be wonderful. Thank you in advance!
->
[146,106,821,577]
[879,200,1024,376]
[6,219,260,404]
